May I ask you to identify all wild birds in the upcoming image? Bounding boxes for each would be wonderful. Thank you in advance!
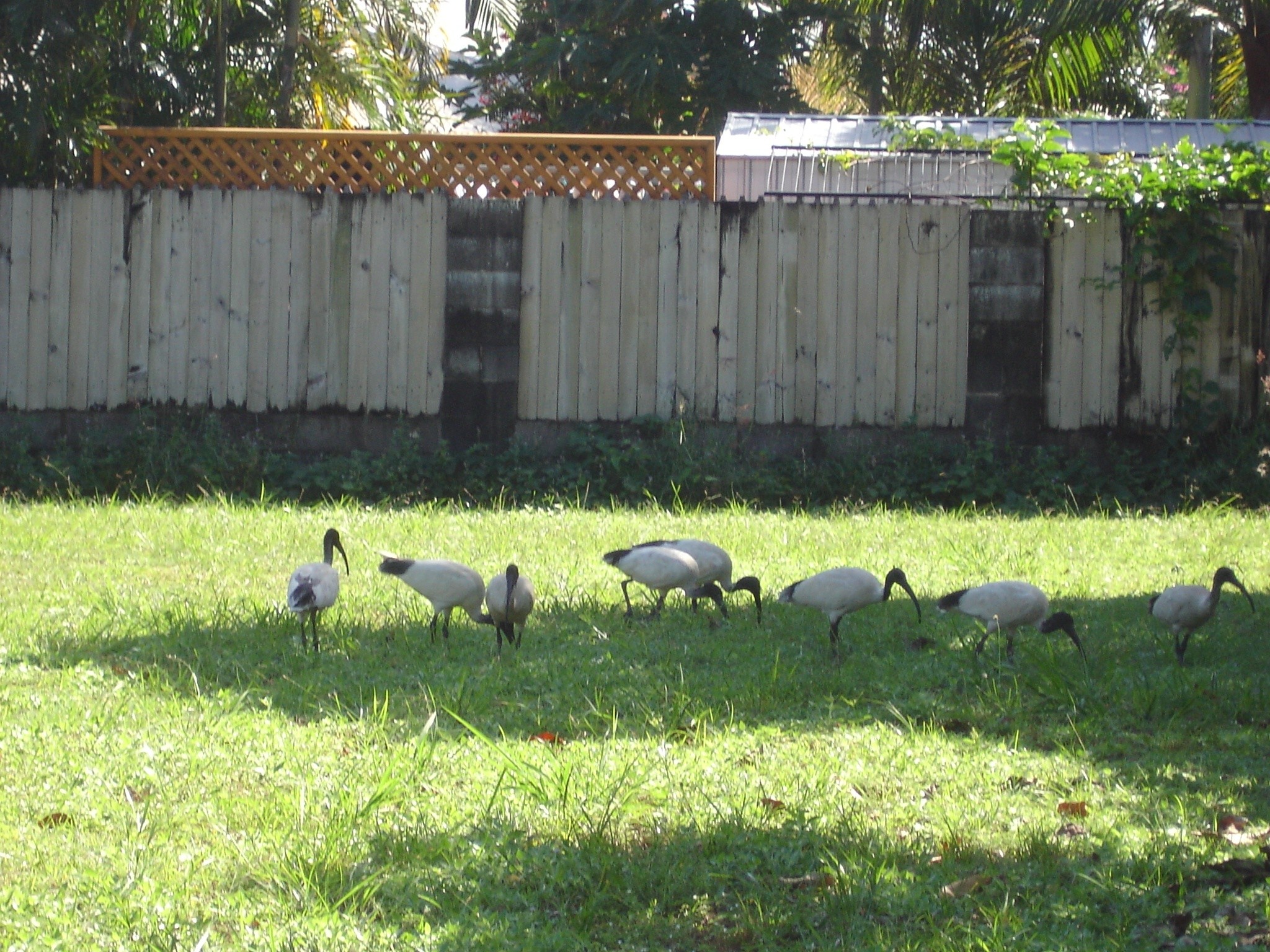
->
[936,581,1088,666]
[779,565,921,660]
[1147,566,1256,667]
[287,528,349,656]
[601,538,763,630]
[376,551,535,653]
